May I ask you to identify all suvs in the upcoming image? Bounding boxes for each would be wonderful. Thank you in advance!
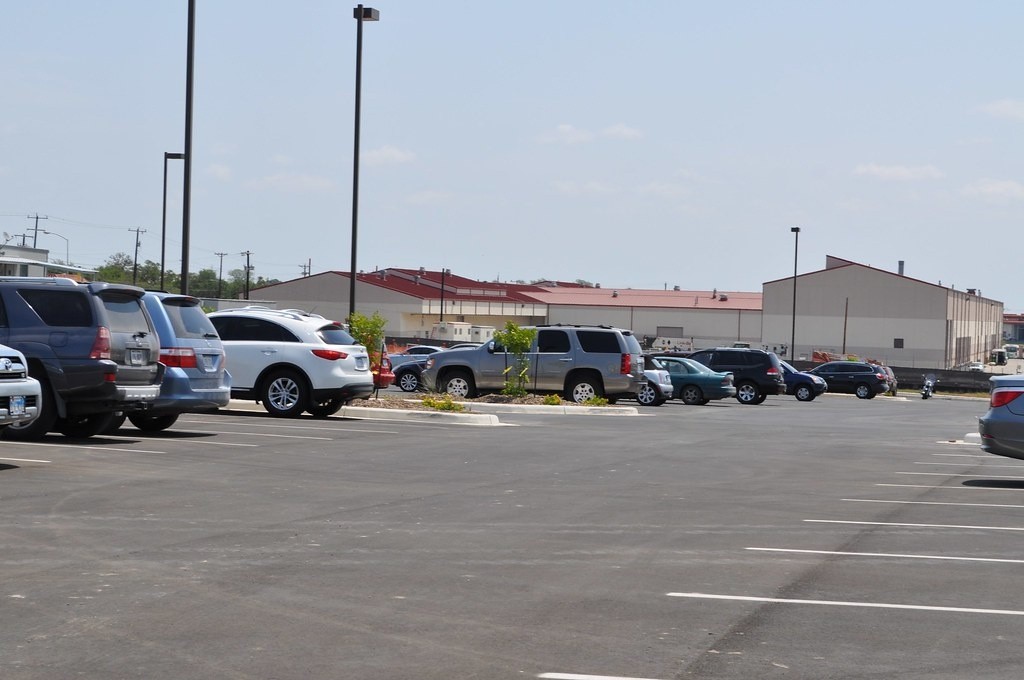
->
[799,361,890,400]
[683,345,788,404]
[0,276,167,443]
[421,323,650,403]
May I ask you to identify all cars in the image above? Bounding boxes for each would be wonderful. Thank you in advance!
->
[779,359,829,402]
[652,356,737,405]
[0,344,43,431]
[353,332,396,389]
[128,289,232,430]
[204,304,376,418]
[967,361,985,374]
[391,343,485,392]
[635,355,674,408]
[979,373,1024,461]
[385,344,446,371]
[880,365,898,397]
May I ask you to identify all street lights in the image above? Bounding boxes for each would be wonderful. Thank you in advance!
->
[789,226,803,367]
[160,152,187,290]
[44,230,69,266]
[347,4,381,338]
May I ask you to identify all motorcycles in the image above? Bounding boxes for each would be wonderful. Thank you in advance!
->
[921,372,941,400]
